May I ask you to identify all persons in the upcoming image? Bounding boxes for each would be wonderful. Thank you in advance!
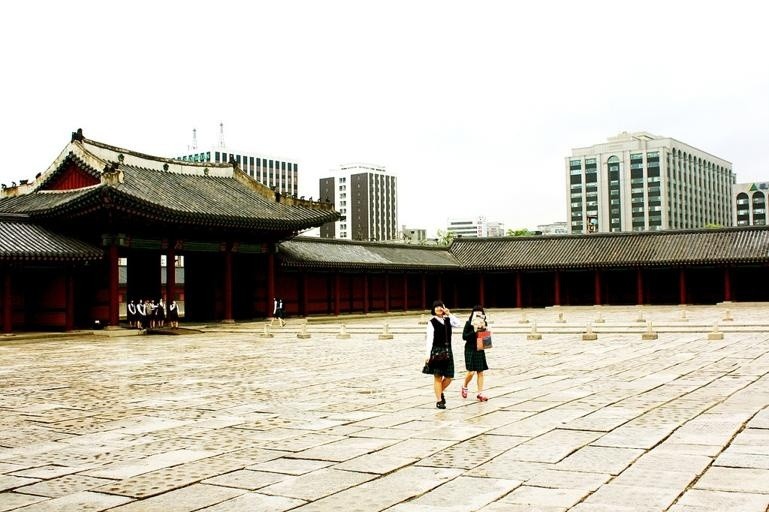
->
[126,299,180,329]
[279,298,287,326]
[420,300,460,410]
[270,297,283,327]
[461,305,489,403]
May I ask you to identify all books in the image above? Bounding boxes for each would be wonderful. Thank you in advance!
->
[477,331,491,338]
[470,312,486,329]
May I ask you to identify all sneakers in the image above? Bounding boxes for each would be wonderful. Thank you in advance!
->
[462,386,468,398]
[436,401,446,409]
[477,393,488,401]
[441,393,446,404]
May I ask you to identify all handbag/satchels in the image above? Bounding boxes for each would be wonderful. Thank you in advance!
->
[476,330,492,352]
[428,352,450,370]
